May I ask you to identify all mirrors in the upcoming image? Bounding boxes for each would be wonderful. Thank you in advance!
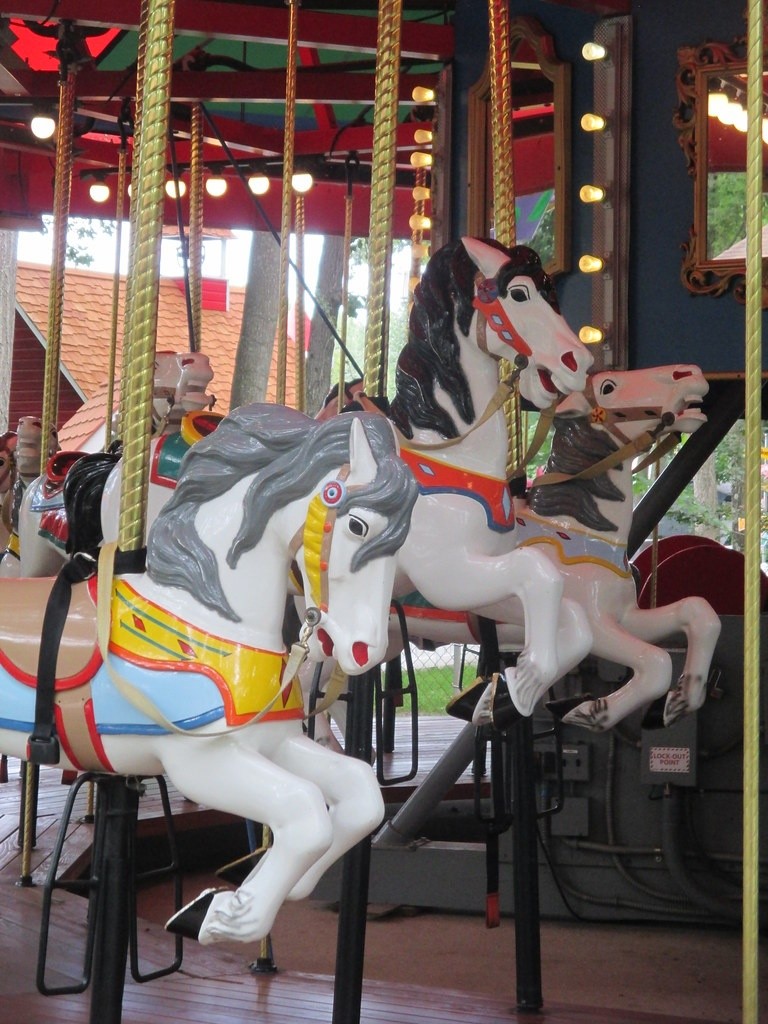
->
[468,14,573,279]
[673,35,768,310]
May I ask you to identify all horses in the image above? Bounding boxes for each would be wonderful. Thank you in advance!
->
[1,236,722,946]
